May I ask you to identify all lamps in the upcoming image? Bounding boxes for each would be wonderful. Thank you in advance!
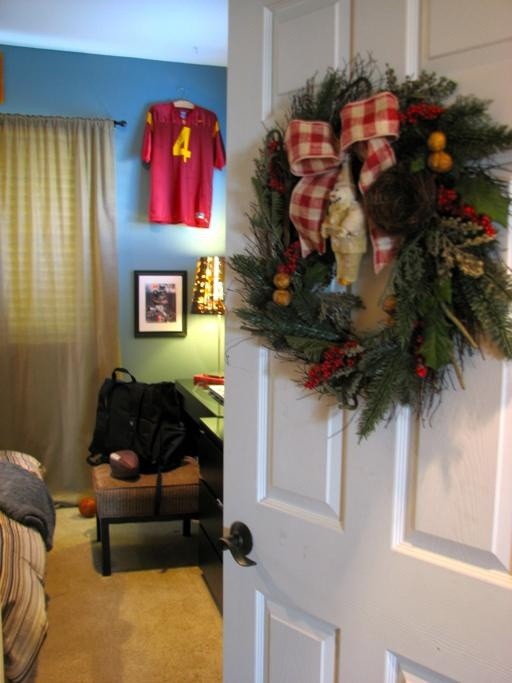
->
[186,252,227,387]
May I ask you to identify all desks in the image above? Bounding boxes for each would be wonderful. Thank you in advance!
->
[170,375,223,615]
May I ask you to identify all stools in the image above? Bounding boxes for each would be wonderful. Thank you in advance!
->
[90,452,200,579]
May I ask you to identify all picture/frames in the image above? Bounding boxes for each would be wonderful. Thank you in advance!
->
[131,268,188,339]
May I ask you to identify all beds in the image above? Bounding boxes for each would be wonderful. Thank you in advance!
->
[1,448,51,683]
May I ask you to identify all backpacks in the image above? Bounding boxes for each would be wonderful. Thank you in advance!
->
[86,366,189,473]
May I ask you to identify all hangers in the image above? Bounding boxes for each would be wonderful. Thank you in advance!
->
[172,86,195,109]
